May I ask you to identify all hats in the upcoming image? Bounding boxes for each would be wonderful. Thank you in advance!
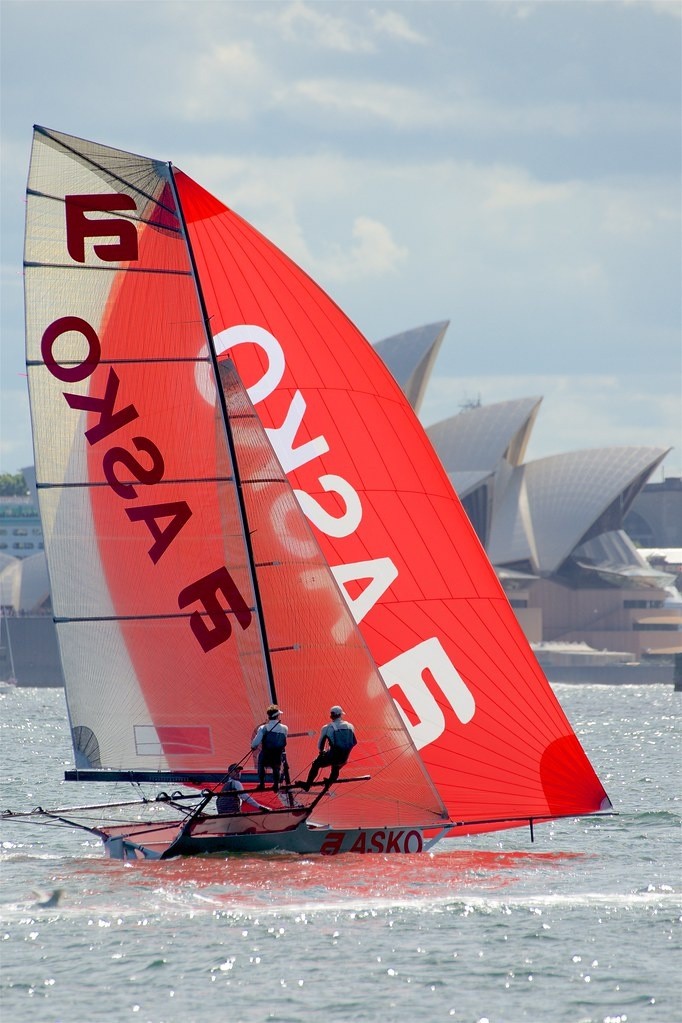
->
[228,764,243,772]
[330,706,347,715]
[267,709,283,718]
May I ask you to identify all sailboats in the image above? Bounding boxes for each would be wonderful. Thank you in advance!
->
[1,122,624,855]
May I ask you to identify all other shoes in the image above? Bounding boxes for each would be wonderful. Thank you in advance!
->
[255,785,264,790]
[270,786,279,791]
[299,780,309,792]
[323,777,329,787]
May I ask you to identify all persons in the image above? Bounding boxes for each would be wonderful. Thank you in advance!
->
[216,763,273,814]
[244,704,289,791]
[295,706,358,793]
